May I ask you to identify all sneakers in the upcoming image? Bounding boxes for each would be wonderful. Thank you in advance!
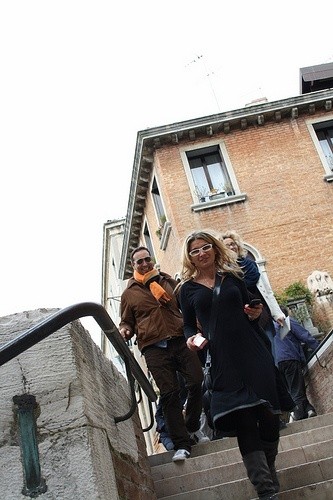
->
[172,449,190,461]
[187,430,210,444]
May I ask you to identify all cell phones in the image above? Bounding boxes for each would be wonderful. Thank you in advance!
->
[248,299,261,308]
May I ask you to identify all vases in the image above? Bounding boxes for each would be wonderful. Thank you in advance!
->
[227,192,232,196]
[209,192,225,199]
[200,197,205,202]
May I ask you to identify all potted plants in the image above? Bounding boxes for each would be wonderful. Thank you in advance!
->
[156,215,166,239]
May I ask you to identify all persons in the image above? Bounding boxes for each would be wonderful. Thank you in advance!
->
[217,229,277,337]
[269,306,320,419]
[119,231,298,500]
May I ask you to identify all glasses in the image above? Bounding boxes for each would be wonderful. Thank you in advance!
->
[133,257,151,264]
[189,244,214,257]
[226,242,235,248]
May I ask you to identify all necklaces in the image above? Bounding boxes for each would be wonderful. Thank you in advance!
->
[207,282,215,290]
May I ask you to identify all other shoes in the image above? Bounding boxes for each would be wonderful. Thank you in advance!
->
[308,410,317,417]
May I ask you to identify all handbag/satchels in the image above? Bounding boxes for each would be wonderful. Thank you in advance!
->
[201,367,214,430]
